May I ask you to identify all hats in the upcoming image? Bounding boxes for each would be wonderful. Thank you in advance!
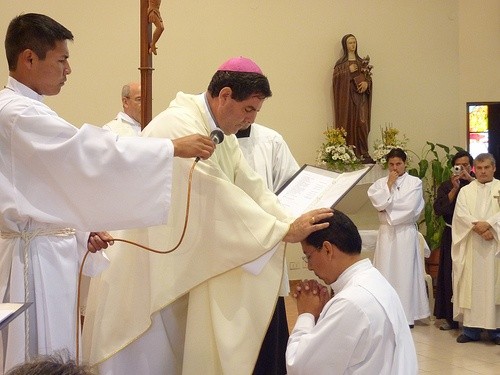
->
[217,56,263,76]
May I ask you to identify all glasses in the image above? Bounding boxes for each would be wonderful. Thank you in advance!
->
[301,246,321,263]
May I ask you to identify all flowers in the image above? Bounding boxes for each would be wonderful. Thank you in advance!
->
[369,122,411,166]
[316,126,364,172]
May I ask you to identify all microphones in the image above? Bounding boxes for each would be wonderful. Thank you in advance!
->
[194,129,224,162]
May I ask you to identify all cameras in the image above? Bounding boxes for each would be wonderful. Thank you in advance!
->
[454,166,463,173]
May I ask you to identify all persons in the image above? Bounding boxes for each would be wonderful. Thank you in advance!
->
[333,34,377,164]
[148,0,165,55]
[433,151,477,330]
[0,12,216,375]
[101,80,142,136]
[81,56,333,375]
[235,123,301,375]
[285,208,419,375]
[367,147,431,328]
[451,153,500,344]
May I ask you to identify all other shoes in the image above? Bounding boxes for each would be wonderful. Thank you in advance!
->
[488,328,500,345]
[439,319,459,330]
[457,328,481,343]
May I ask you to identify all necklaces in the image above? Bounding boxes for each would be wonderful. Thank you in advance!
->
[393,175,406,190]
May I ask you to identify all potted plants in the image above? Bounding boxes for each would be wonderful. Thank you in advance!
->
[403,138,469,289]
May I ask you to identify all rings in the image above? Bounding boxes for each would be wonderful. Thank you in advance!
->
[311,217,316,223]
